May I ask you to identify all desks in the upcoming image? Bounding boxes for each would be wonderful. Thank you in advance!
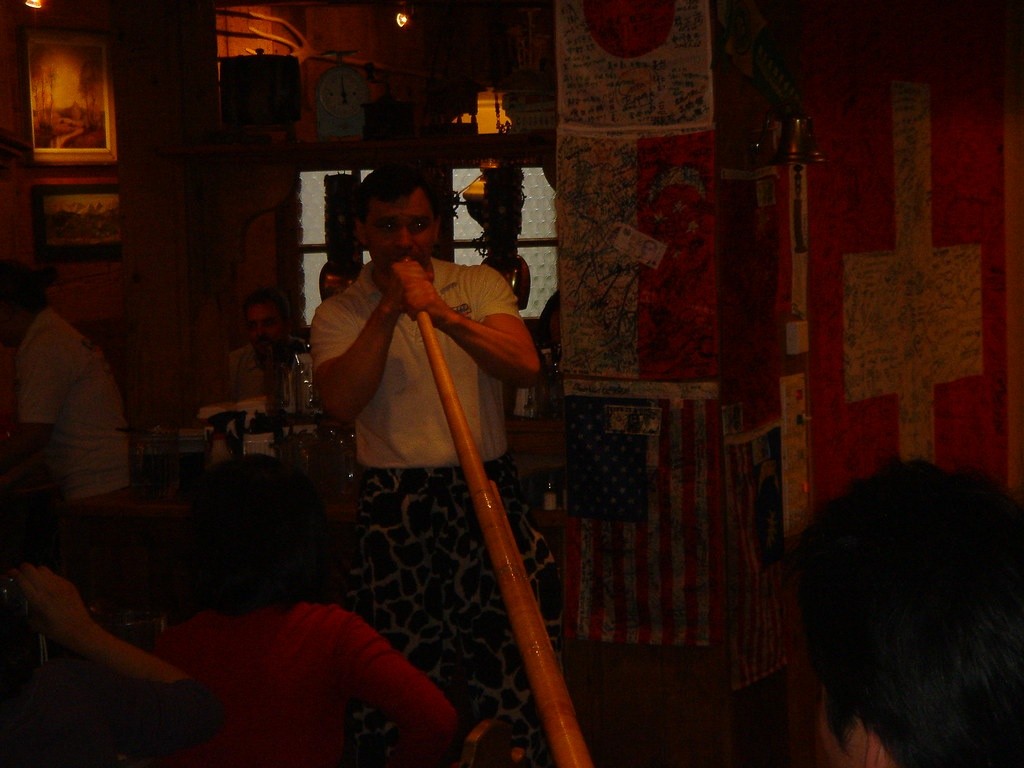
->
[61,483,564,651]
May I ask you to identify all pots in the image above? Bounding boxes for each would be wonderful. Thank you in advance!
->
[219,48,301,124]
[361,99,414,137]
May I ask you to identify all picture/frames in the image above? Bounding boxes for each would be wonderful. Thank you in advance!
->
[16,24,122,168]
[32,184,124,262]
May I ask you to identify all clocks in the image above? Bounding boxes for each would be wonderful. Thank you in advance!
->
[313,65,372,142]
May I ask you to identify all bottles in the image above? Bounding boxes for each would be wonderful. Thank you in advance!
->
[543,468,563,510]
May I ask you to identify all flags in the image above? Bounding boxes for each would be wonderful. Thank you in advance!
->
[724,428,797,683]
[555,127,723,382]
[556,0,713,127]
[561,381,723,644]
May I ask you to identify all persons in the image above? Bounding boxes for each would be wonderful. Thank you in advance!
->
[223,289,307,402]
[0,562,226,768]
[794,462,1024,768]
[0,259,130,560]
[149,453,456,768]
[308,162,564,768]
